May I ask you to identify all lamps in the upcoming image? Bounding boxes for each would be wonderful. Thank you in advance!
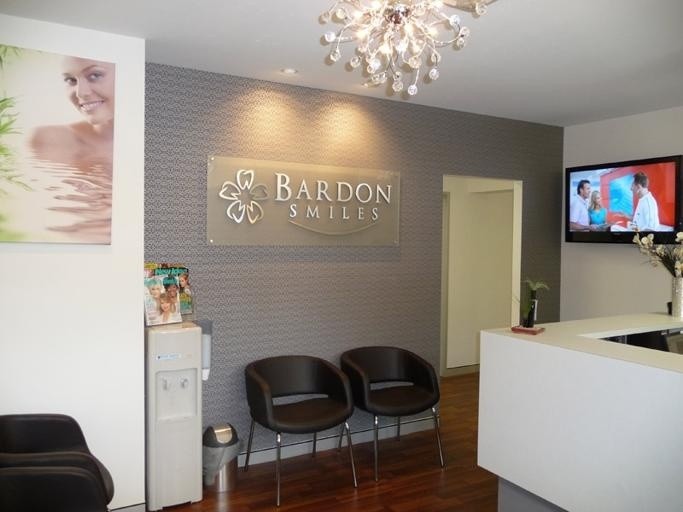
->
[320,0,493,95]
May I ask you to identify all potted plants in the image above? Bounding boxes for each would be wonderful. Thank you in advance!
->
[522,278,549,328]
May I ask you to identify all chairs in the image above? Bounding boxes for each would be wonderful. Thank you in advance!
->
[340,346,445,482]
[0,414,114,505]
[0,465,108,512]
[244,353,357,506]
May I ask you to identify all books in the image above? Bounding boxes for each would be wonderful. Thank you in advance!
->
[143,261,193,327]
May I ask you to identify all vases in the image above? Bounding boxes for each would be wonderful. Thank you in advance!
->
[671,276,683,317]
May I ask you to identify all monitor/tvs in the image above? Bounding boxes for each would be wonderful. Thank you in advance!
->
[566,154,682,244]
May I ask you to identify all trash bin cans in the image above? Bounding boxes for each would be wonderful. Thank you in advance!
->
[202,422,242,495]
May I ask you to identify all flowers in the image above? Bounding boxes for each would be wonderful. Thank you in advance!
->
[632,231,683,276]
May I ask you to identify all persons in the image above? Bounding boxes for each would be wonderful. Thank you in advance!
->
[28,54,115,160]
[570,179,598,231]
[629,171,660,231]
[588,190,608,232]
